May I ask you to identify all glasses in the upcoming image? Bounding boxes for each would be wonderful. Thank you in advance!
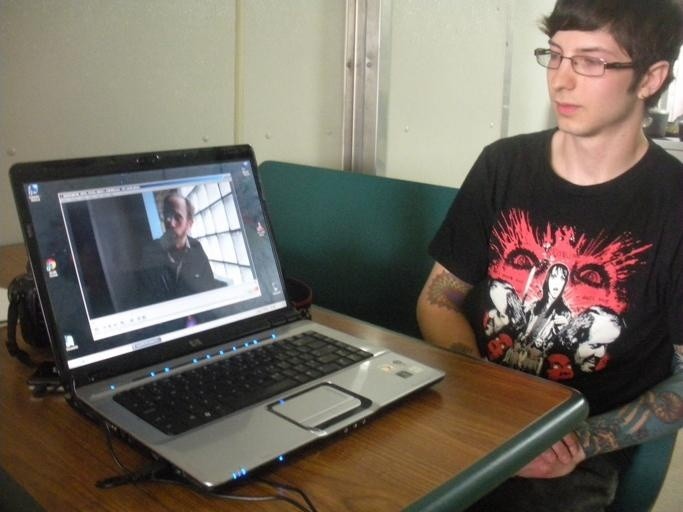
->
[535,49,633,77]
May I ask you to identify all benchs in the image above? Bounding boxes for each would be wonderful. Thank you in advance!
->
[259,161,683,512]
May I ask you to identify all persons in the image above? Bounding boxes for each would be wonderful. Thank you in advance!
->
[526,263,571,343]
[413,0,683,512]
[137,194,215,307]
[483,333,515,361]
[544,352,577,381]
[486,279,524,331]
[563,304,625,368]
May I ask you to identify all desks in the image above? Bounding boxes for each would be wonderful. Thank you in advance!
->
[0,239,590,512]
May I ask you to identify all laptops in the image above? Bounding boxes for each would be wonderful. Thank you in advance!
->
[8,145,447,496]
